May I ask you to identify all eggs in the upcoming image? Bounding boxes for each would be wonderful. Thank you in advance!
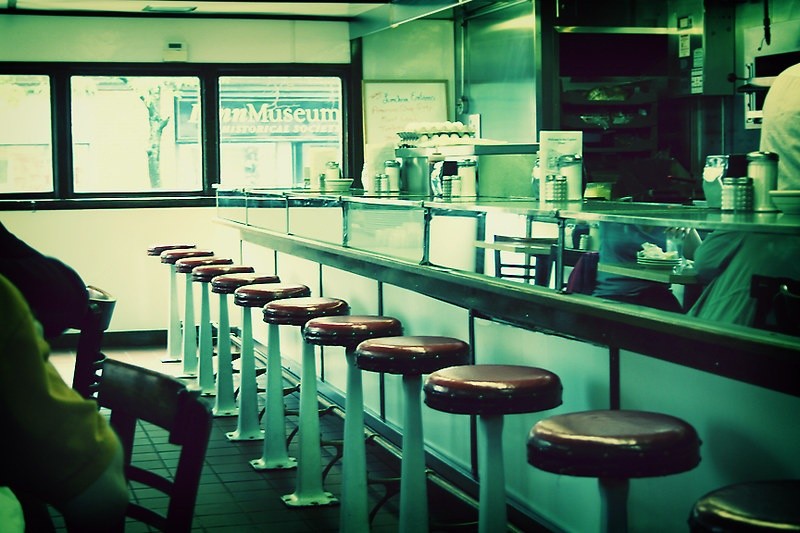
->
[404,121,470,141]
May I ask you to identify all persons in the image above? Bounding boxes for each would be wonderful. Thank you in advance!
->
[760,62,800,191]
[591,221,800,327]
[0,220,127,533]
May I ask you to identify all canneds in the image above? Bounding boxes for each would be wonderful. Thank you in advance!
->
[579,234,591,251]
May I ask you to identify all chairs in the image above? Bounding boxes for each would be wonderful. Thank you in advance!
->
[44,284,214,533]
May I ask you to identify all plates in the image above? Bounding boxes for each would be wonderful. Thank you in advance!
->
[636,256,695,273]
[693,201,706,206]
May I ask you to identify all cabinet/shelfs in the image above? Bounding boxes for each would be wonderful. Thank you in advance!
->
[554,79,659,176]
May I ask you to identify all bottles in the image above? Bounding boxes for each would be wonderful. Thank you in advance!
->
[304,161,340,189]
[532,152,583,202]
[563,220,600,250]
[374,160,400,193]
[720,150,779,212]
[441,156,477,197]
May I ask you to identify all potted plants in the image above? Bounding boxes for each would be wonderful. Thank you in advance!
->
[325,162,354,192]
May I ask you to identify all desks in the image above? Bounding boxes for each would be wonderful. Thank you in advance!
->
[593,262,702,284]
[472,242,551,255]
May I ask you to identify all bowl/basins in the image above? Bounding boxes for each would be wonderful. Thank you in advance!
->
[768,190,800,215]
[325,178,354,191]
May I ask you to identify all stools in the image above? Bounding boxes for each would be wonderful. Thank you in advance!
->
[145,244,800,533]
